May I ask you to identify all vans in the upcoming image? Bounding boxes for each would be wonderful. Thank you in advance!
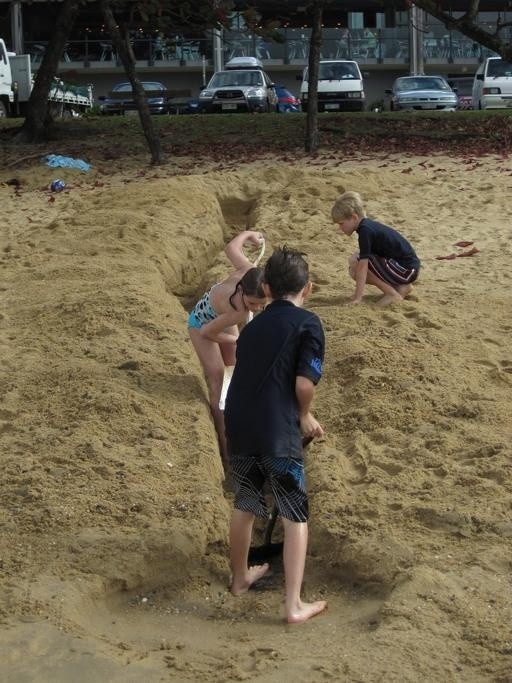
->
[298,58,367,112]
[471,53,512,110]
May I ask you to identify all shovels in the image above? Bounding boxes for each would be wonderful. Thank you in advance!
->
[249,436,313,561]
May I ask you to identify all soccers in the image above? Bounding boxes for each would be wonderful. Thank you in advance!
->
[51,179,63,191]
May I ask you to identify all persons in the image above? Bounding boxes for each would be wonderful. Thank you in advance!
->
[154,32,167,52]
[223,248,331,624]
[331,190,421,308]
[187,231,278,462]
[340,29,354,52]
[361,28,377,52]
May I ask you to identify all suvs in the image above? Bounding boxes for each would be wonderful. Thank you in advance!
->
[197,55,280,114]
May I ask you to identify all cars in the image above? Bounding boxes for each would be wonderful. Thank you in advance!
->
[95,81,172,115]
[432,77,475,110]
[168,95,199,112]
[380,74,460,111]
[272,82,301,113]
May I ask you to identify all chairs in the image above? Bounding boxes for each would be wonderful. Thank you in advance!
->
[32,35,497,59]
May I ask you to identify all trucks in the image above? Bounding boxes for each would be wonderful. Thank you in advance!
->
[0,37,96,119]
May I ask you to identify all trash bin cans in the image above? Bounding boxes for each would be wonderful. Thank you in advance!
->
[384,89,393,112]
